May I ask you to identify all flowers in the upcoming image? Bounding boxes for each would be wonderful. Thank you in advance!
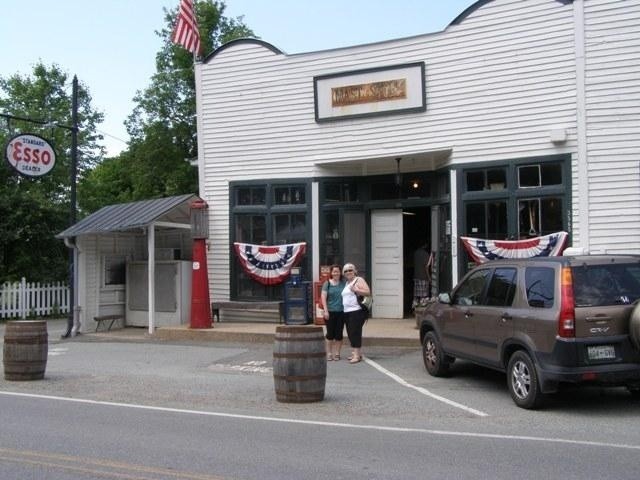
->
[413,298,436,308]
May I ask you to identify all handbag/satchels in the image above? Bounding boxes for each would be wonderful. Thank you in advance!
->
[319,297,324,310]
[360,295,373,310]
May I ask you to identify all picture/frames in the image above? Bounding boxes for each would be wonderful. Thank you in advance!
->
[312,61,427,123]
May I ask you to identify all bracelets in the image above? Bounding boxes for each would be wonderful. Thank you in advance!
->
[323,310,328,313]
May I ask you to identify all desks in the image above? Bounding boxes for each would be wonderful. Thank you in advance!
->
[279,302,286,324]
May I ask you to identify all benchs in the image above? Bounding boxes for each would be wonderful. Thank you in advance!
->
[94,314,124,333]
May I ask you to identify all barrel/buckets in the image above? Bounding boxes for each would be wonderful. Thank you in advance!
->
[272,325,327,403]
[3,320,49,381]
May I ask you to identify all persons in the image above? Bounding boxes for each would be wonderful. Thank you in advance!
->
[412,241,430,331]
[340,263,370,363]
[320,265,347,361]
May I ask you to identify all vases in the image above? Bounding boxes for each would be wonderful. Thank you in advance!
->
[416,310,427,327]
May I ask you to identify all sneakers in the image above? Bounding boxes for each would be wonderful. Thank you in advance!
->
[347,352,363,364]
[327,354,341,362]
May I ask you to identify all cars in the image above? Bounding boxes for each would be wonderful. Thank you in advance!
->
[419,254,640,410]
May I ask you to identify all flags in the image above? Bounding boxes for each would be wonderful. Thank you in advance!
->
[170,0,202,56]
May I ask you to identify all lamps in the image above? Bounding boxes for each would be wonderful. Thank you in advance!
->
[394,159,403,186]
